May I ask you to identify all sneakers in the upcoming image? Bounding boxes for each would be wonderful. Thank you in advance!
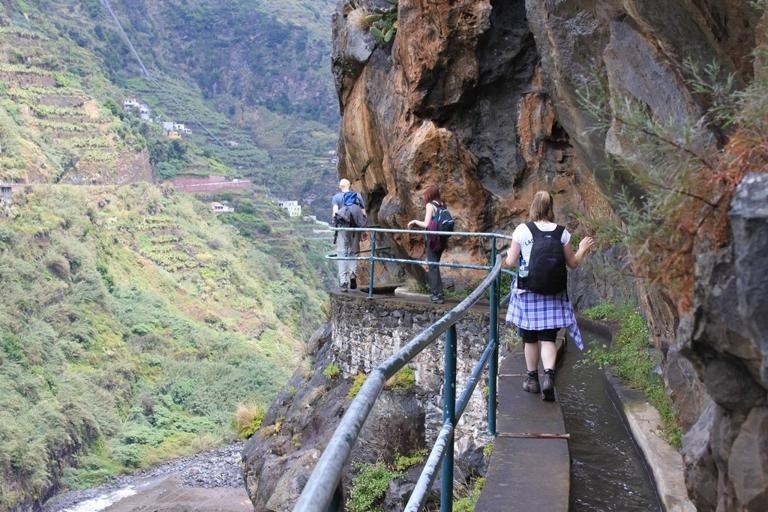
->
[522,377,540,394]
[350,274,357,289]
[429,295,444,304]
[338,286,348,293]
[541,374,556,401]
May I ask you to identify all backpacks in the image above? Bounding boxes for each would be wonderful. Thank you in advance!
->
[516,221,568,295]
[333,194,368,228]
[430,200,455,238]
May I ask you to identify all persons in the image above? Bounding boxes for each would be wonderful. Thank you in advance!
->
[504,189,596,405]
[406,184,455,305]
[330,178,369,292]
